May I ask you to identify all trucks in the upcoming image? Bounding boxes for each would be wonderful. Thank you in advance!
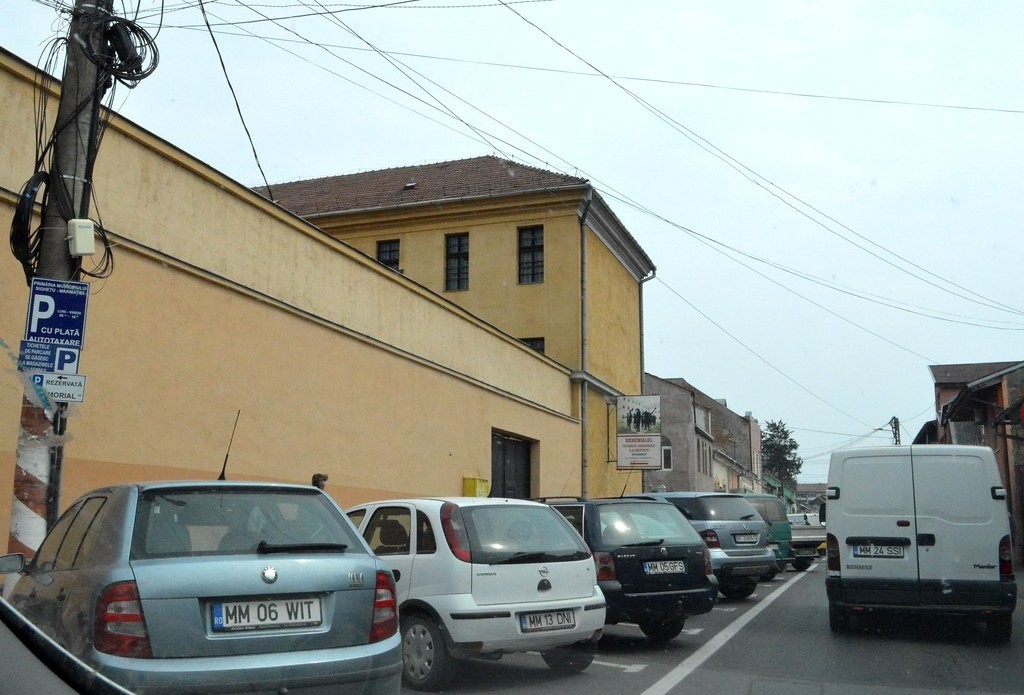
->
[790,523,826,570]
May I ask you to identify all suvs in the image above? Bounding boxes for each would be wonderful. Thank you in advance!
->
[530,494,719,641]
[650,493,776,602]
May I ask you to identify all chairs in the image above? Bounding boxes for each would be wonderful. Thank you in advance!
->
[148,521,534,555]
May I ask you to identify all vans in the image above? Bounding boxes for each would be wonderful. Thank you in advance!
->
[786,513,819,524]
[744,493,793,581]
[819,444,1018,643]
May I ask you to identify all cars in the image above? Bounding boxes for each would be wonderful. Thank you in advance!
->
[310,496,607,691]
[0,478,403,695]
